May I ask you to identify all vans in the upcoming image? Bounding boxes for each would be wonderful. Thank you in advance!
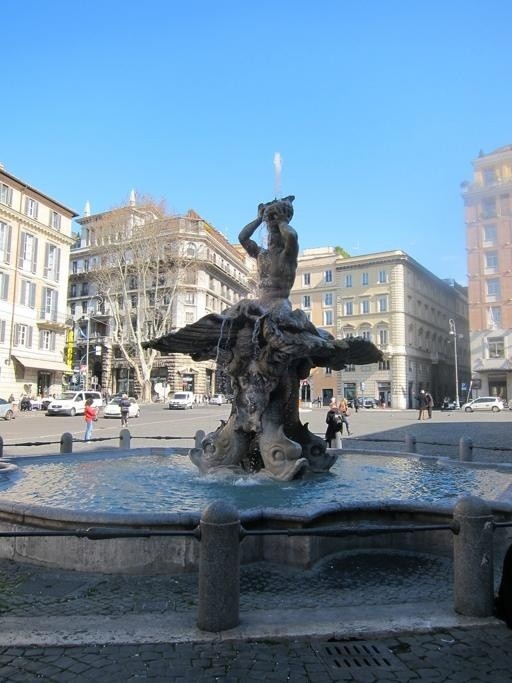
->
[169,391,194,409]
[461,396,503,412]
[48,390,104,416]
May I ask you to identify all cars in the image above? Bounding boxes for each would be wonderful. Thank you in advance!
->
[507,399,512,410]
[209,393,225,405]
[1,397,20,420]
[104,392,141,417]
[447,398,464,409]
[348,395,380,408]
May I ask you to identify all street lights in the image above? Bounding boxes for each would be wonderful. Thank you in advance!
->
[84,293,103,390]
[448,318,460,409]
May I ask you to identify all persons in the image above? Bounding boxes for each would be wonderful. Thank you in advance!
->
[354,395,360,412]
[425,391,434,419]
[8,394,14,403]
[318,393,322,408]
[83,398,96,443]
[339,398,354,436]
[381,398,385,409]
[325,402,349,449]
[119,393,131,428]
[238,202,299,314]
[417,389,428,420]
